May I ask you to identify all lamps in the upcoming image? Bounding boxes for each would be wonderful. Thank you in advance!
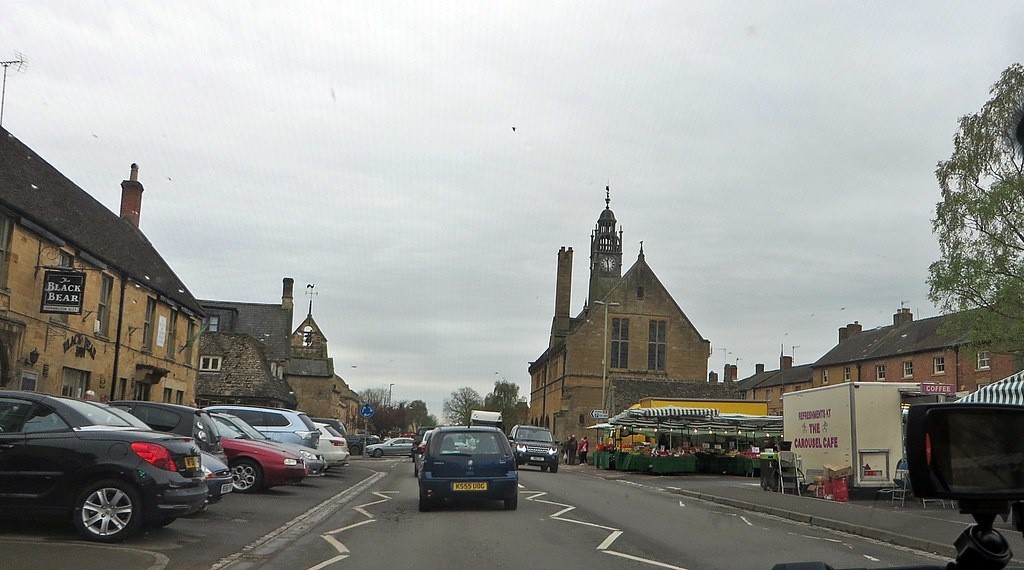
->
[24,347,40,367]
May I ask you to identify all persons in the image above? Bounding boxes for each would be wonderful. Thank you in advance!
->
[84,390,110,403]
[658,433,668,450]
[562,434,589,465]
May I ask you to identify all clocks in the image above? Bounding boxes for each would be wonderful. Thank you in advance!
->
[600,255,616,273]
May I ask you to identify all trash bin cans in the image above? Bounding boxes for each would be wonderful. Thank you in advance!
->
[760,453,780,490]
[814,476,848,500]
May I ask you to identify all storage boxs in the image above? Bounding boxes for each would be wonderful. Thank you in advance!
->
[805,462,854,499]
[759,453,778,459]
[603,429,656,452]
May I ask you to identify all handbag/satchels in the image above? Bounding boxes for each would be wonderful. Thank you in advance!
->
[578,449,583,455]
[563,452,567,460]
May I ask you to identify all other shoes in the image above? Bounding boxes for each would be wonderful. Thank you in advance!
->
[586,463,588,466]
[580,463,585,466]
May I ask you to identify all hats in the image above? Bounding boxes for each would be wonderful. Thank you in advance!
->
[572,435,575,438]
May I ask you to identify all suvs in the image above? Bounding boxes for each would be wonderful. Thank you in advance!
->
[102,398,230,468]
[508,424,560,473]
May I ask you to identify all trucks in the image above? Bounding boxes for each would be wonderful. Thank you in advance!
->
[469,410,505,447]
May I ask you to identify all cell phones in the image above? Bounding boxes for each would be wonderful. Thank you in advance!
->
[905,402,1024,501]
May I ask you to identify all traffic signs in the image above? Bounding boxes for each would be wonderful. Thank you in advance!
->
[589,409,609,419]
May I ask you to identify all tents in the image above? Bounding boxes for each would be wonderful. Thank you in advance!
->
[955,369,1024,406]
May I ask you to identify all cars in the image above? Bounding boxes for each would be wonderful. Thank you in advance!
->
[0,388,208,542]
[189,404,378,494]
[200,451,234,503]
[411,427,487,476]
[366,437,415,458]
[415,424,519,510]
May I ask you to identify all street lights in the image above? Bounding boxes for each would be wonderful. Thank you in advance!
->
[389,383,395,407]
[593,300,620,409]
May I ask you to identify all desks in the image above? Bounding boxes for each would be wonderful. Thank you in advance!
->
[593,451,761,478]
[760,458,779,492]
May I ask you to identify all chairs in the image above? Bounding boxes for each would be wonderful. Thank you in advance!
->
[473,436,495,451]
[527,433,534,438]
[440,436,456,450]
[518,430,526,438]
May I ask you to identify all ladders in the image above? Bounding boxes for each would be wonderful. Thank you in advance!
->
[891,458,921,507]
